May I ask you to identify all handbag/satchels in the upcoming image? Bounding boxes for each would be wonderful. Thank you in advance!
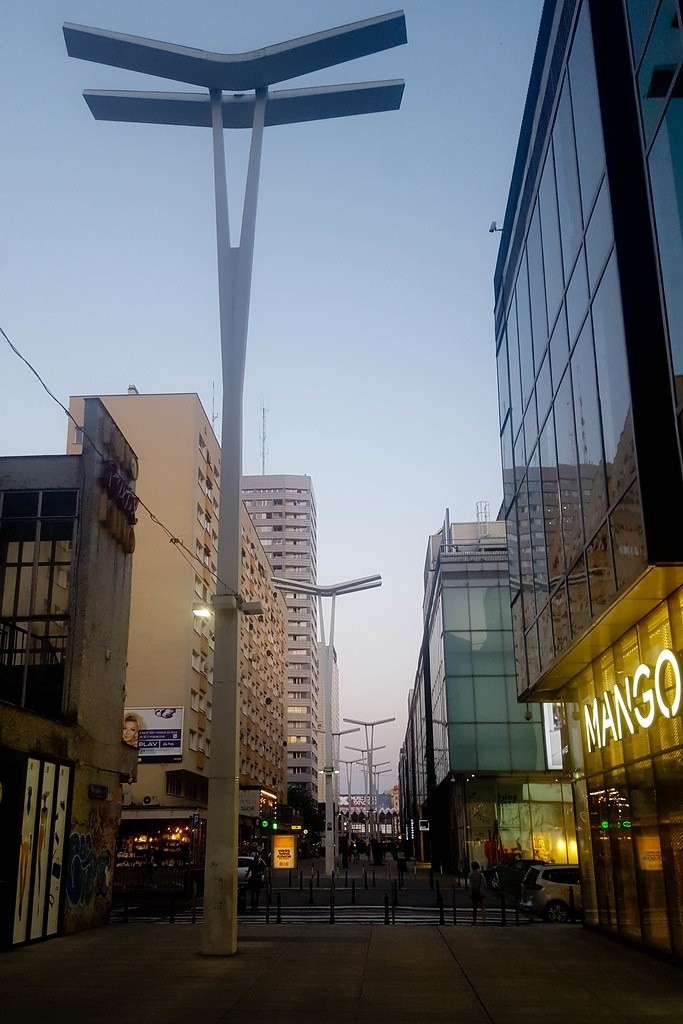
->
[469,887,484,908]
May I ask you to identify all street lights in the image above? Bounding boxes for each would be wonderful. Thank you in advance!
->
[342,717,395,845]
[311,728,358,876]
[62,9,410,956]
[270,574,383,875]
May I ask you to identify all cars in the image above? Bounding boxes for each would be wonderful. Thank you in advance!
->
[238,857,269,886]
[519,864,583,923]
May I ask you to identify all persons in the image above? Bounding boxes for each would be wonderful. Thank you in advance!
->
[123,712,146,748]
[349,838,394,864]
[245,855,266,912]
[469,861,487,925]
